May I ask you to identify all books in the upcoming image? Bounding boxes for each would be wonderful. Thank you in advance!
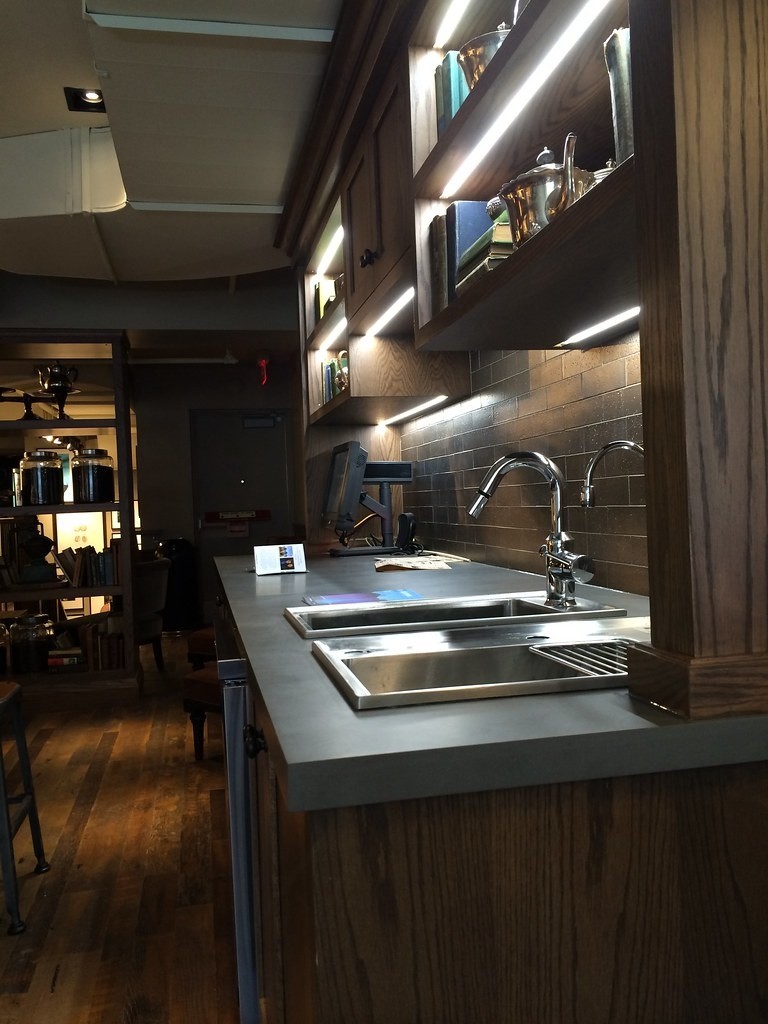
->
[373,554,463,571]
[46,636,107,672]
[52,545,115,587]
[301,589,423,607]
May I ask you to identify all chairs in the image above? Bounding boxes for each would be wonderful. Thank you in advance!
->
[135,557,172,673]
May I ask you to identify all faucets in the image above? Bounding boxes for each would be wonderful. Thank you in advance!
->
[579,439,646,508]
[464,451,595,607]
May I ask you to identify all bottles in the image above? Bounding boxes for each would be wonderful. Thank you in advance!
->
[71,448,115,504]
[0,623,10,677]
[9,612,54,673]
[19,451,64,507]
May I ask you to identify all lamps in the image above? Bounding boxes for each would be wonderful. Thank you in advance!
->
[0,359,82,422]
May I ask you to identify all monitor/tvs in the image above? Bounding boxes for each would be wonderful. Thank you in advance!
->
[321,441,366,529]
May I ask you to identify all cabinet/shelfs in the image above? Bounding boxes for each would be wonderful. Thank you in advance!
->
[411,0,653,354]
[218,609,768,1024]
[292,180,476,428]
[0,328,145,715]
[330,51,416,338]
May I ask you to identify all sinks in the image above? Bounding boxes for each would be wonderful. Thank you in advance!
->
[284,598,561,640]
[311,637,627,711]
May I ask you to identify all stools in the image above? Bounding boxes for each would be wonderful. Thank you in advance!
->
[188,627,217,671]
[0,681,53,937]
[182,668,221,761]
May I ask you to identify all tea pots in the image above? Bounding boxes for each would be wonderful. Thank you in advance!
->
[497,132,596,252]
[457,0,520,92]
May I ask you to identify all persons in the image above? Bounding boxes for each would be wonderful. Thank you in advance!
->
[280,547,293,569]
[16,513,69,631]
